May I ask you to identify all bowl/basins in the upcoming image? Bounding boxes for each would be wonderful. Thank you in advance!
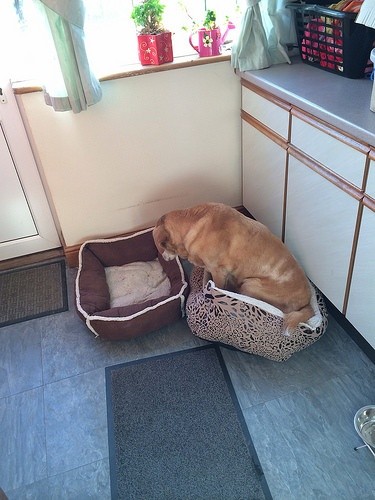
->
[354,405,375,449]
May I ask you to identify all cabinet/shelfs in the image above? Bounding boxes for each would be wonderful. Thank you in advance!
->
[234,54,375,365]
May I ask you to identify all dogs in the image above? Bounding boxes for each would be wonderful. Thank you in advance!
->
[152,202,315,337]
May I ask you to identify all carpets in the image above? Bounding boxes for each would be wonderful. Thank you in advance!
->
[0,259,69,328]
[105,343,274,500]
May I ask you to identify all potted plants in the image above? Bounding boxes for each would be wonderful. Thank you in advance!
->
[130,0,173,66]
[189,9,236,57]
